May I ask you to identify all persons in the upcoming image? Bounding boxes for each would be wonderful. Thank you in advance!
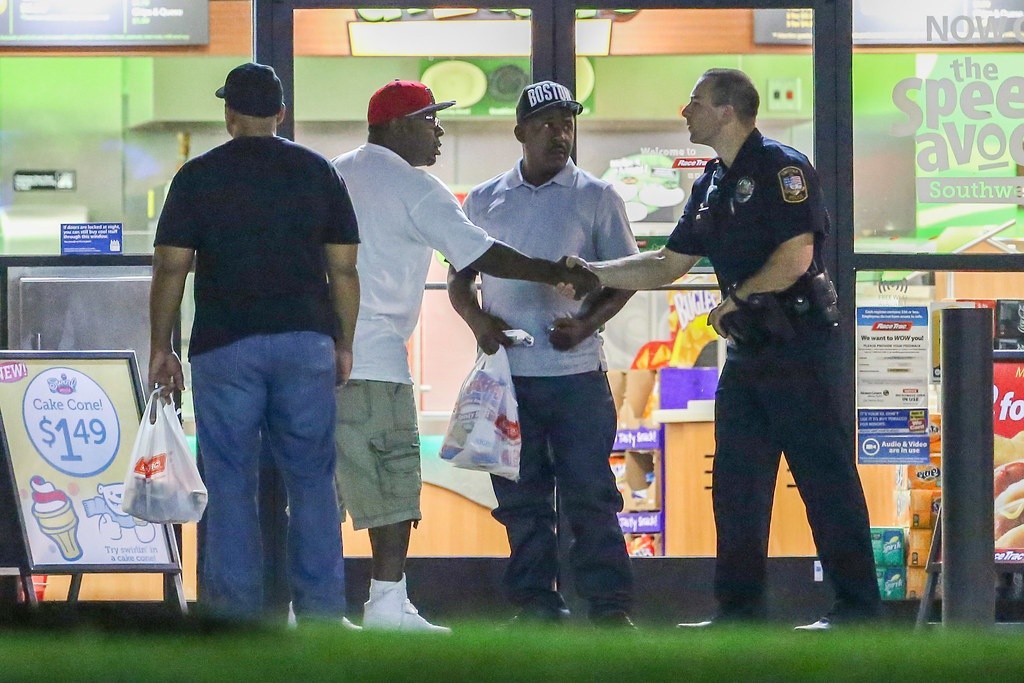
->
[148,63,361,629]
[287,78,600,632]
[550,68,883,631]
[447,82,640,632]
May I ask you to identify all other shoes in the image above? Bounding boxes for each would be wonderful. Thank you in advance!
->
[588,609,640,629]
[512,607,573,619]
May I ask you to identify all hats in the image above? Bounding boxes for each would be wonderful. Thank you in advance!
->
[515,81,583,125]
[215,63,283,117]
[368,79,457,124]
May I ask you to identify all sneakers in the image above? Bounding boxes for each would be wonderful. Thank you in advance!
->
[289,600,362,631]
[363,574,453,632]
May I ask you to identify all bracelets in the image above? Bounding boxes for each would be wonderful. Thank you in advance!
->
[729,285,750,307]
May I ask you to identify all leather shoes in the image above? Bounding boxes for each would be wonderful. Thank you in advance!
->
[676,621,770,630]
[794,619,879,630]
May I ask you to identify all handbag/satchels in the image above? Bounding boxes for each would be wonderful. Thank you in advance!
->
[122,386,208,524]
[440,329,533,482]
